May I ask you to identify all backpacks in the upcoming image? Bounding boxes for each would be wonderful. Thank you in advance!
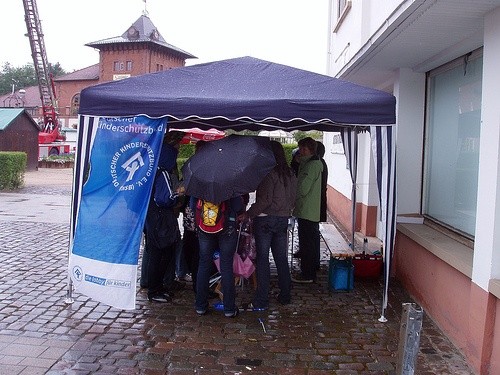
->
[194,197,230,235]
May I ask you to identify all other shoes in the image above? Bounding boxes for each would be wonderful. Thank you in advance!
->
[224,308,239,317]
[194,308,207,314]
[292,249,302,258]
[147,296,168,303]
[290,273,314,284]
[176,273,193,282]
[275,292,290,305]
[238,301,269,311]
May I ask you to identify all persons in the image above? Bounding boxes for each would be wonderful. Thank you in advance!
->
[190,195,245,317]
[140,136,329,302]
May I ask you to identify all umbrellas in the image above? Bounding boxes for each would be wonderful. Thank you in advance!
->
[181,134,278,204]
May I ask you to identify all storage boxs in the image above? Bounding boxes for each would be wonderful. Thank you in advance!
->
[328,254,355,290]
[352,254,382,281]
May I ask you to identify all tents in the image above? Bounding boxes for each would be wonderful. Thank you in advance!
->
[66,57,397,323]
